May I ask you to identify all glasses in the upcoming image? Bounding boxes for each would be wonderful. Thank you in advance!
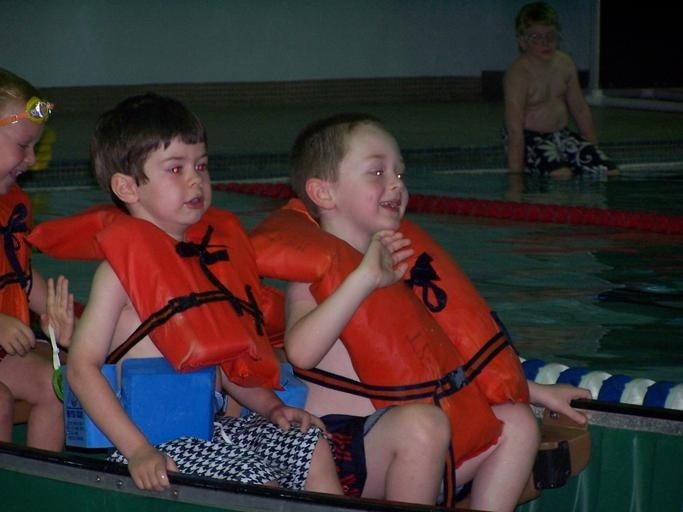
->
[0,95,54,129]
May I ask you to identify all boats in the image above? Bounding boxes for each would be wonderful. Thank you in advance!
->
[0,396,682,512]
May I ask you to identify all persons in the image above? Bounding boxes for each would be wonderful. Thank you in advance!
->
[64,91,345,497]
[280,111,592,512]
[504,0,619,177]
[0,64,80,453]
